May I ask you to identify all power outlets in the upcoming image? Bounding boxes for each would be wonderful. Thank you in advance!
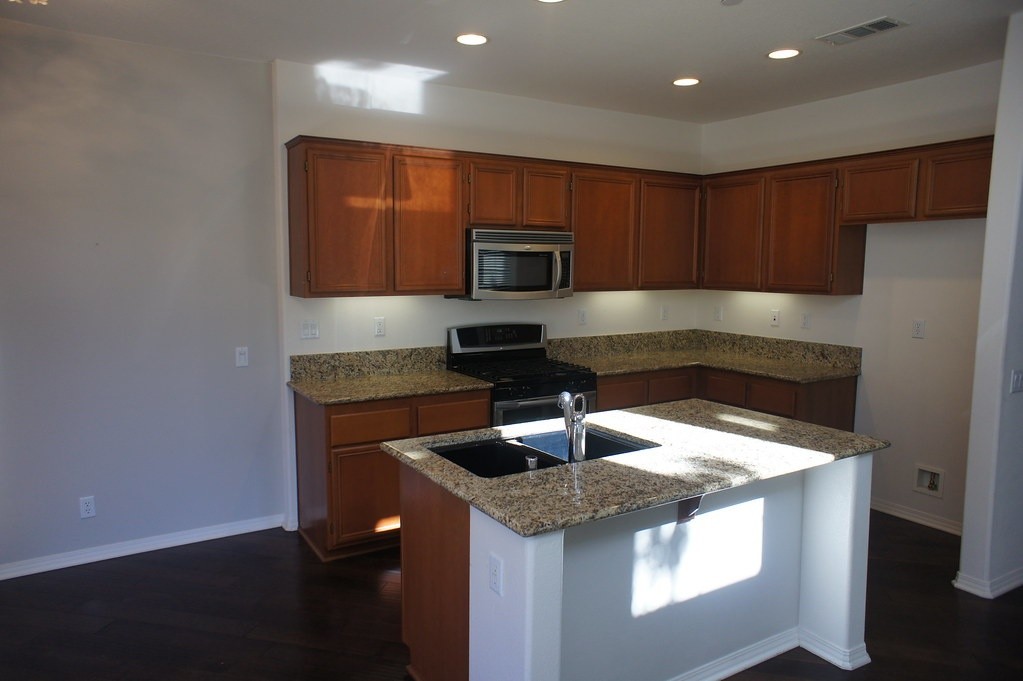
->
[80,496,97,519]
[800,311,810,329]
[911,318,925,340]
[374,316,385,337]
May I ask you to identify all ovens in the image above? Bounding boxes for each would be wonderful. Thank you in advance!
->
[493,391,596,428]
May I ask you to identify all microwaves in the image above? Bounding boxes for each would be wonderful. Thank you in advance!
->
[444,229,574,301]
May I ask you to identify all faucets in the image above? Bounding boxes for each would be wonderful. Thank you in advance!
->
[556,392,585,463]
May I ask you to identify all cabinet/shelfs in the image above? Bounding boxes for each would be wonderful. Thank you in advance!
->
[292,372,860,562]
[284,134,996,301]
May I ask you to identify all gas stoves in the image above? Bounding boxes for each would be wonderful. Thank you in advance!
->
[448,322,596,401]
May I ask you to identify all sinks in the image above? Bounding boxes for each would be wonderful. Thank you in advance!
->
[419,438,563,478]
[511,421,662,463]
